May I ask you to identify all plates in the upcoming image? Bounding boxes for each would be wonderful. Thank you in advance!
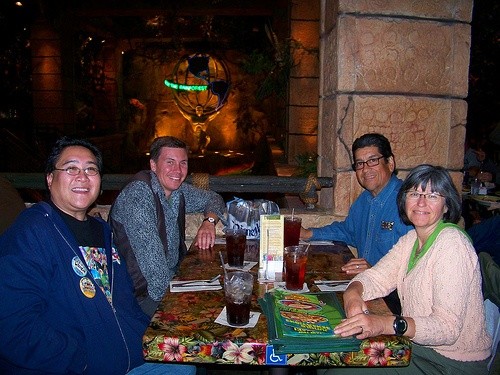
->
[462,191,470,195]
[473,196,500,205]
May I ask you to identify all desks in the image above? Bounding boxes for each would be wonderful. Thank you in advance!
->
[460,192,500,226]
[144,234,411,375]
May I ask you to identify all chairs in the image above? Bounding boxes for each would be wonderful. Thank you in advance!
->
[478,252,500,375]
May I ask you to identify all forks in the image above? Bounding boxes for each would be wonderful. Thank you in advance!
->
[173,274,221,285]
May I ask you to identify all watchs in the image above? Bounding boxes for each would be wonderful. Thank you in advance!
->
[203,218,217,225]
[393,315,408,337]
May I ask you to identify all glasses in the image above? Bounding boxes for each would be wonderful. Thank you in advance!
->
[352,155,387,171]
[404,191,447,200]
[54,167,101,176]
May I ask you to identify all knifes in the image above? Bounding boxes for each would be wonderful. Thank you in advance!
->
[172,283,224,288]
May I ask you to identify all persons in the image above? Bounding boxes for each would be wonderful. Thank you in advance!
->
[298,133,416,274]
[462,126,500,265]
[107,136,225,320]
[0,138,196,375]
[316,164,493,375]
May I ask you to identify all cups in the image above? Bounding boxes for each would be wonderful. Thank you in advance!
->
[284,246,308,292]
[223,272,254,325]
[470,177,480,195]
[284,215,301,247]
[226,229,246,267]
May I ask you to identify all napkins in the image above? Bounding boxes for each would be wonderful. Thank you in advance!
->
[170,279,222,293]
[299,240,336,246]
[214,305,262,328]
[314,280,350,292]
